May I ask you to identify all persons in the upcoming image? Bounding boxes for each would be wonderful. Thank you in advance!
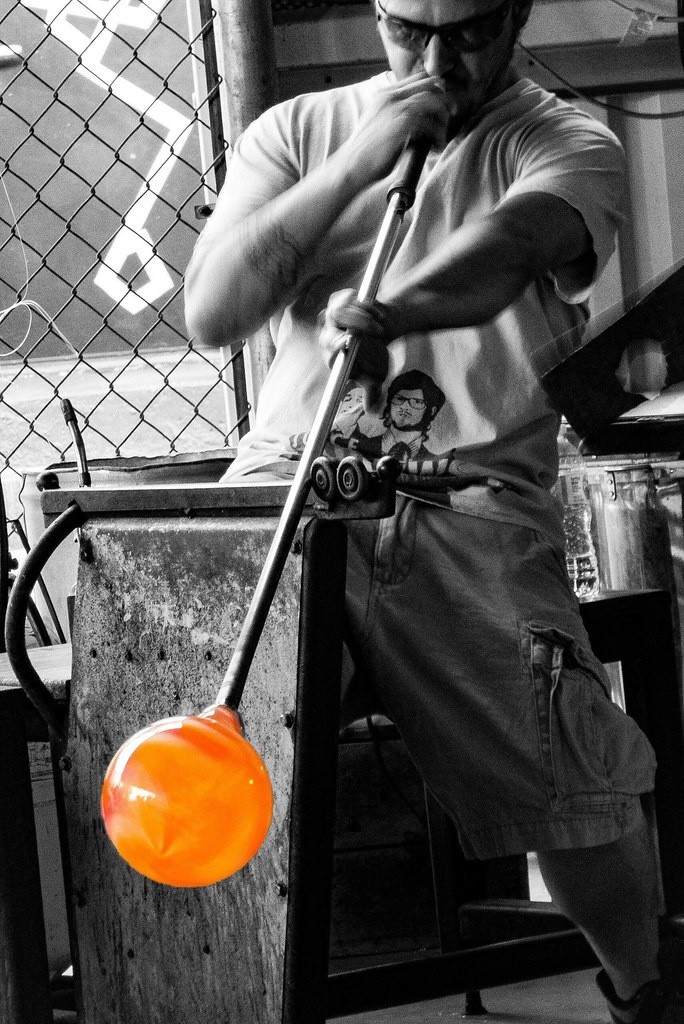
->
[181,0,684,1024]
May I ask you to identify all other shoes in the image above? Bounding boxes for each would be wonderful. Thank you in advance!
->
[596,968,684,1024]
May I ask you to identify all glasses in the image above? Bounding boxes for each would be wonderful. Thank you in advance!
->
[376,1,509,55]
